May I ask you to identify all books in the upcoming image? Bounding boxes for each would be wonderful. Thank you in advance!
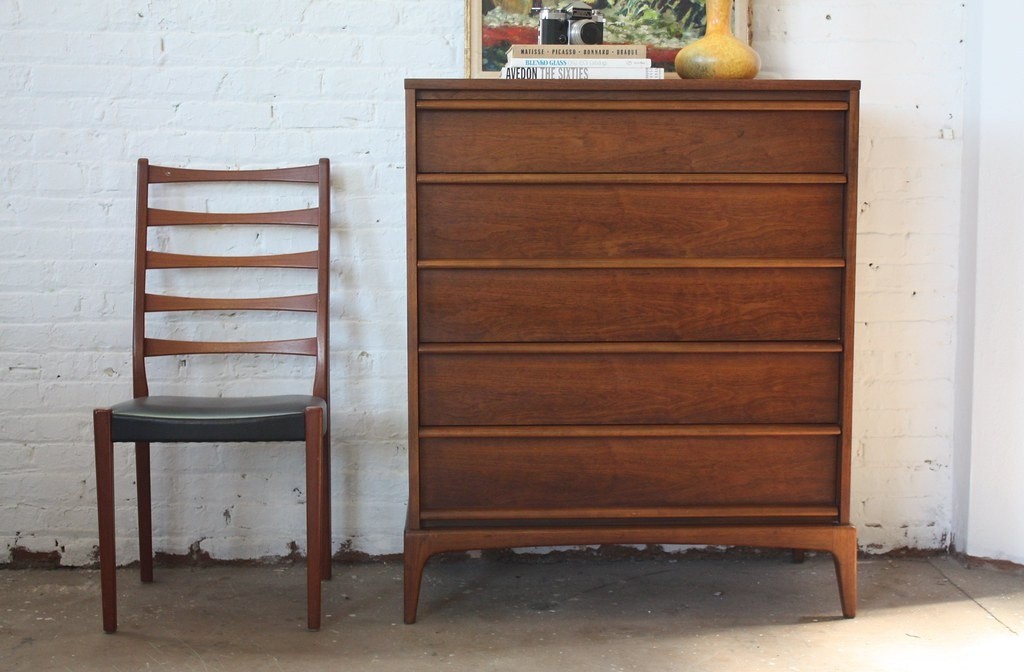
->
[499,44,665,80]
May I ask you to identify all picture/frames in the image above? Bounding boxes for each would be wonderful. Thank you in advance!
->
[465,0,754,79]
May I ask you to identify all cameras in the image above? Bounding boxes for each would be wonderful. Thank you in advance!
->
[538,2,604,45]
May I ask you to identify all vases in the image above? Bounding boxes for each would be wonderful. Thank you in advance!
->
[675,0,760,80]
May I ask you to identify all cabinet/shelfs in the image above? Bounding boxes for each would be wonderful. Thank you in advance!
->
[404,78,860,624]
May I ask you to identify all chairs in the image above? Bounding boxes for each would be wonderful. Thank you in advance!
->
[95,158,331,630]
